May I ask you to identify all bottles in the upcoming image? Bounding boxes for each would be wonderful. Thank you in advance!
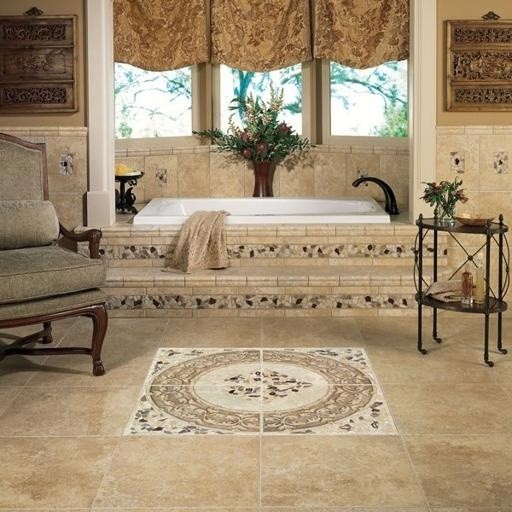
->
[474,258,486,304]
[461,263,473,305]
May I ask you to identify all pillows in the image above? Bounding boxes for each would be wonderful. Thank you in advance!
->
[0,200,59,250]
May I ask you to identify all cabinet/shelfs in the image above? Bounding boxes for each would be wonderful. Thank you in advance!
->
[414,214,510,367]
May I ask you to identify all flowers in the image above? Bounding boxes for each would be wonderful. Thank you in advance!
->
[192,83,328,174]
[418,176,470,219]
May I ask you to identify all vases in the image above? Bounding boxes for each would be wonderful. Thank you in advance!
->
[438,204,456,223]
[252,161,276,198]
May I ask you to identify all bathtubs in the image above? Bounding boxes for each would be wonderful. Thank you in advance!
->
[133,196,393,224]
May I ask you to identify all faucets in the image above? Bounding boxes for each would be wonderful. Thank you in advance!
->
[352,176,399,216]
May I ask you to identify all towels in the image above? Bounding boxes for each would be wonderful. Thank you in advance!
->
[161,210,232,273]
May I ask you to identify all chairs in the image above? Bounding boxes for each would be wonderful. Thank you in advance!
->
[0,132,110,375]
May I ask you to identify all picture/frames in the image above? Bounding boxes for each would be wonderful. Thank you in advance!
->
[446,19,512,112]
[0,14,78,114]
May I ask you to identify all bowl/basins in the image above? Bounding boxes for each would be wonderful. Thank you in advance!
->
[456,212,496,226]
